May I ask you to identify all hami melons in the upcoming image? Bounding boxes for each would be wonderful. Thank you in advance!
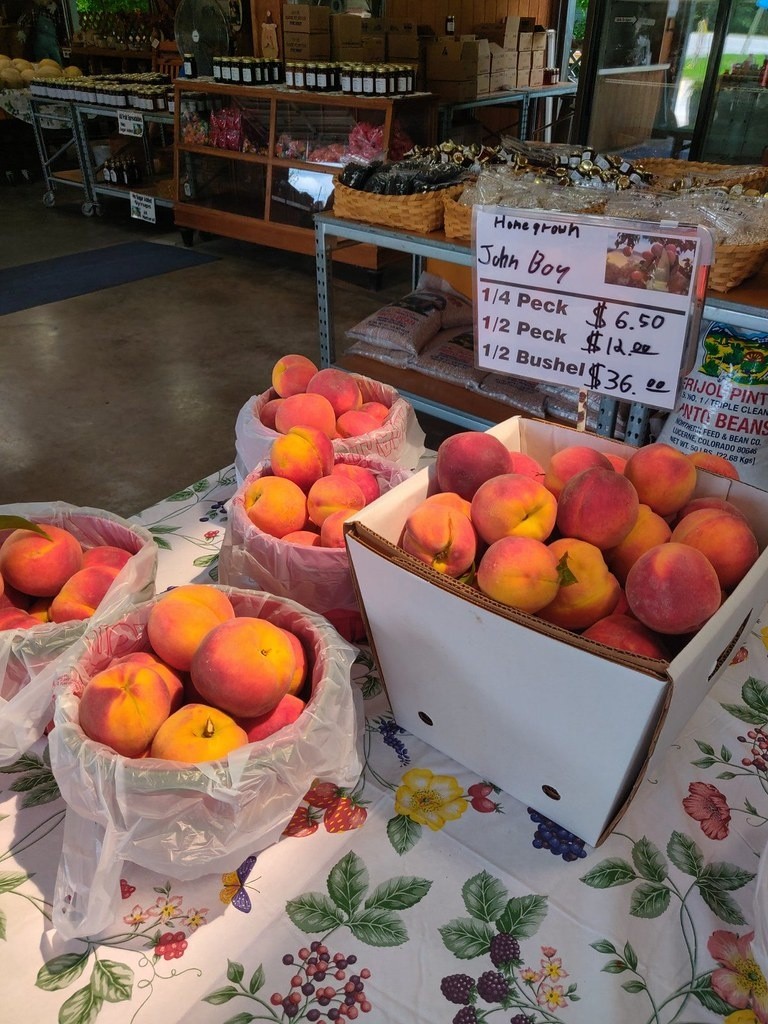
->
[0,54,83,87]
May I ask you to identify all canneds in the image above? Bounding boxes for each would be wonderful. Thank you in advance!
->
[212,56,415,97]
[445,15,455,35]
[542,68,559,85]
[29,72,224,116]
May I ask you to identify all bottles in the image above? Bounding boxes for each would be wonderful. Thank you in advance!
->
[102,153,146,188]
[543,68,560,85]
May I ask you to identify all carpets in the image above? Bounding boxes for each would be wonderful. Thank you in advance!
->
[0,239,224,317]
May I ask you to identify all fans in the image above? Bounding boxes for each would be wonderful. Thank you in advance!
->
[175,0,245,77]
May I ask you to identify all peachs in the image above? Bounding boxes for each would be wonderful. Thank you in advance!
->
[400,432,758,664]
[77,583,312,764]
[0,522,136,630]
[622,243,676,280]
[243,422,381,549]
[260,355,391,443]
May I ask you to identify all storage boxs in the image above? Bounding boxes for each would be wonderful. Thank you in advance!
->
[386,33,419,59]
[478,16,522,52]
[386,14,418,35]
[329,11,362,48]
[329,45,363,63]
[532,24,550,51]
[360,37,385,61]
[517,17,536,52]
[530,69,544,87]
[283,4,329,33]
[284,31,329,60]
[517,70,530,88]
[517,51,532,70]
[532,51,544,69]
[425,39,517,100]
[342,415,768,849]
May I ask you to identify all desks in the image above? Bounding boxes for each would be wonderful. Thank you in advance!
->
[0,459,768,1024]
[468,82,578,143]
[424,90,527,149]
[58,44,159,77]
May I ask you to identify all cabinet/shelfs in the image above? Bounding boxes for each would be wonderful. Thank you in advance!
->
[173,77,432,291]
[25,93,186,217]
[312,207,768,436]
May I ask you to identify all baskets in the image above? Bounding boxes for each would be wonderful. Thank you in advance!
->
[441,184,609,242]
[632,157,768,191]
[512,141,597,174]
[332,169,467,233]
[604,191,768,293]
